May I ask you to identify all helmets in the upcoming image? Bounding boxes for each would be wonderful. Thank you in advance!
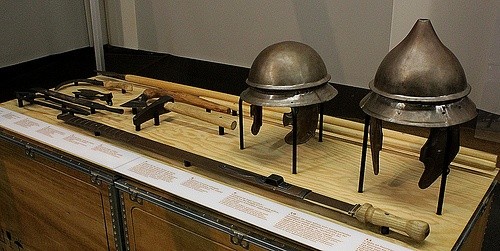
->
[240,40,338,145]
[358,18,478,189]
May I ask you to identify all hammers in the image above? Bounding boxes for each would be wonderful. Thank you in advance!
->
[132,94,237,131]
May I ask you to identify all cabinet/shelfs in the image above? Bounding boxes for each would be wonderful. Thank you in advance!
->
[0,75,500,251]
